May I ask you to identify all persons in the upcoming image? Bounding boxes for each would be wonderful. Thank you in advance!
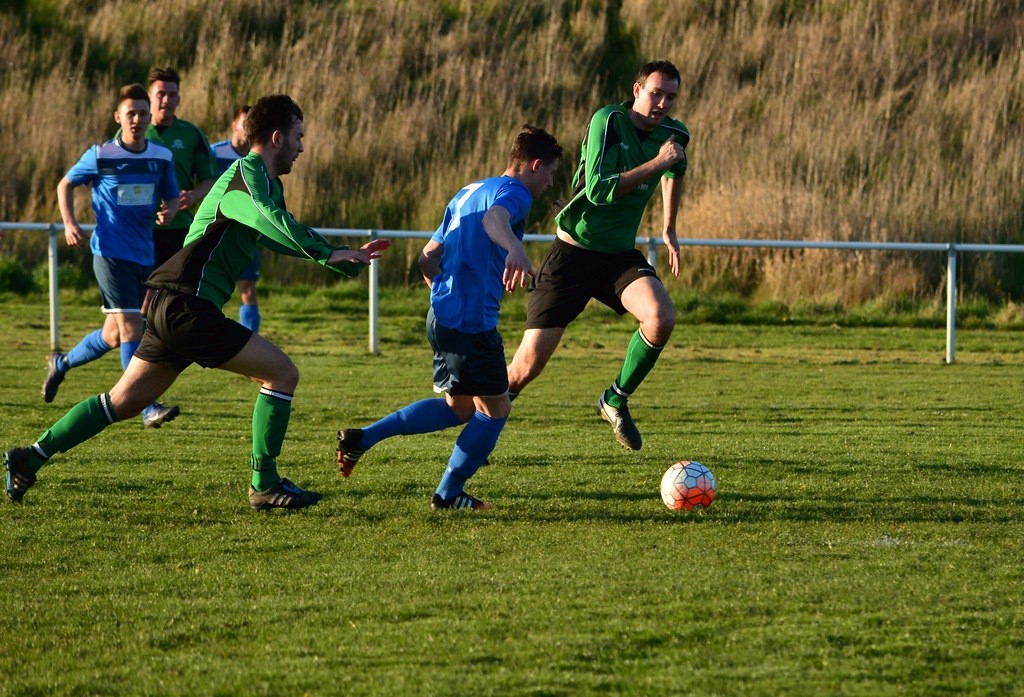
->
[335,125,562,510]
[506,58,690,450]
[4,95,389,509]
[41,66,261,427]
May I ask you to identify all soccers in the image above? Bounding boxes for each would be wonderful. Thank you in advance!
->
[660,460,716,510]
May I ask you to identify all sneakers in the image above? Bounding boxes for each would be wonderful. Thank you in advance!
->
[597,389,643,451]
[40,352,65,403]
[336,428,365,477]
[2,447,37,503]
[431,491,493,511]
[143,405,180,429]
[248,477,323,512]
